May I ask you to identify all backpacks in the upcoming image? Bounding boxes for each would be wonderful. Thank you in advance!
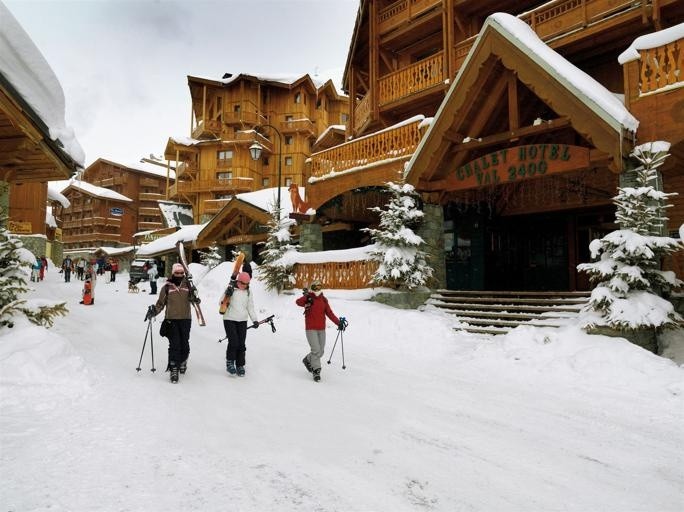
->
[155,274,159,279]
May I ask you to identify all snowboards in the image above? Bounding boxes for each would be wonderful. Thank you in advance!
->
[84,274,91,305]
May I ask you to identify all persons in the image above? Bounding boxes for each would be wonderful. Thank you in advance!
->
[242,259,252,281]
[296,280,342,383]
[31,255,159,305]
[148,262,197,384]
[219,272,259,376]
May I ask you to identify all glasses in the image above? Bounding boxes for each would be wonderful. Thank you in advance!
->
[241,282,249,285]
[174,272,184,274]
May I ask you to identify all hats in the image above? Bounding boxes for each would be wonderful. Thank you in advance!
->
[172,263,184,275]
[237,272,251,283]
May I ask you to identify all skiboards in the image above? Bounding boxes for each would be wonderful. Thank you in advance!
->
[176,241,206,327]
[219,252,245,315]
[302,276,311,315]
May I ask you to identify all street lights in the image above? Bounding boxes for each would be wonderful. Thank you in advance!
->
[249,124,282,218]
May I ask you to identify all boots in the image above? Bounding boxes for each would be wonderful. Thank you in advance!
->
[226,360,245,374]
[303,357,321,381]
[170,361,187,381]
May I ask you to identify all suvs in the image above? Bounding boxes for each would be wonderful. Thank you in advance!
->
[129,259,163,283]
